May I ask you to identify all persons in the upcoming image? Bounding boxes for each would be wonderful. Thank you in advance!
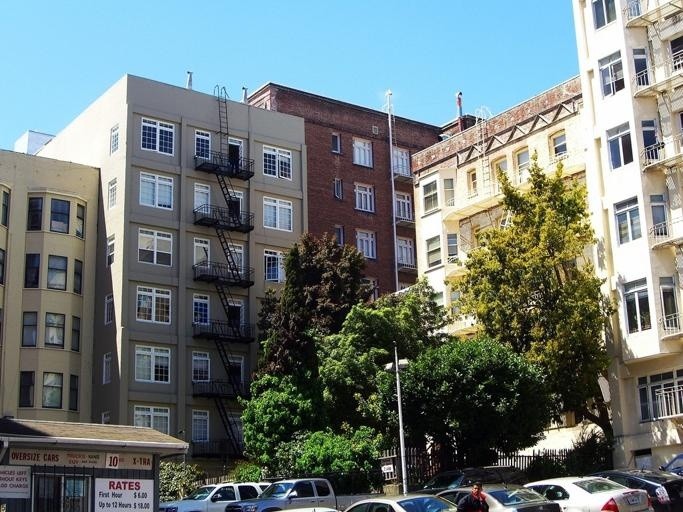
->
[456,482,489,512]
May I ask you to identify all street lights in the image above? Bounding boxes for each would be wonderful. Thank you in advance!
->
[384,339,411,497]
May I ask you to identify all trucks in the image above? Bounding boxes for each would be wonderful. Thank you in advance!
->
[154,478,286,511]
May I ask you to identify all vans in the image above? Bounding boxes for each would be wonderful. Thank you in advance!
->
[416,462,532,496]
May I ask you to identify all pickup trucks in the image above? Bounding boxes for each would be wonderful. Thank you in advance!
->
[222,476,384,512]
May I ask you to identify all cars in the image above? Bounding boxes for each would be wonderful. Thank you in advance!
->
[434,481,563,512]
[585,467,682,511]
[657,452,682,476]
[341,492,461,511]
[520,474,656,511]
[272,505,342,512]
